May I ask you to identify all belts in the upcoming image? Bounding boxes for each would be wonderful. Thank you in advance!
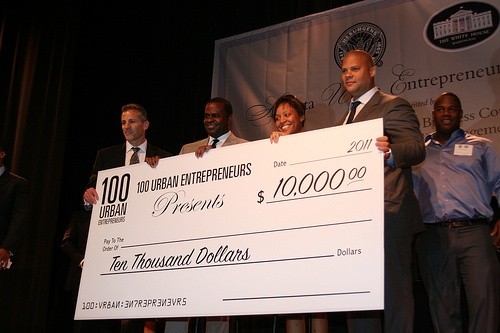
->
[440,219,482,227]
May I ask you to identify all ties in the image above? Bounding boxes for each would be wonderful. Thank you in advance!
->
[130,147,140,164]
[211,138,219,148]
[347,101,361,123]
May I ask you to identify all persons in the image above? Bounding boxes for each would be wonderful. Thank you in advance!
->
[59,208,92,333]
[164,96,253,333]
[330,49,427,333]
[82,102,169,333]
[269,94,329,333]
[407,90,500,333]
[0,150,29,333]
